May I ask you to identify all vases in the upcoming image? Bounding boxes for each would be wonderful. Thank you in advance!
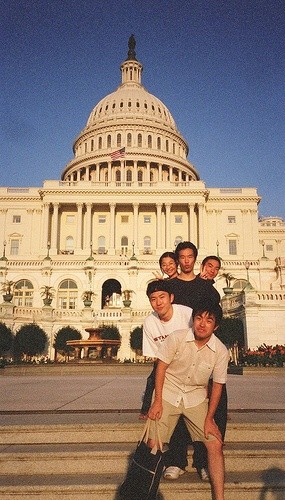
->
[265,362,270,367]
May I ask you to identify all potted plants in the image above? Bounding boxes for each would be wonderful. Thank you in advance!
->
[81,289,99,306]
[121,290,136,307]
[38,284,56,305]
[0,279,21,302]
[216,272,237,295]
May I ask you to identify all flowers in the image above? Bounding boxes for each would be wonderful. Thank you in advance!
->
[239,341,285,364]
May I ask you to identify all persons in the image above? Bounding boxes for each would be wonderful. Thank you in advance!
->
[164,241,226,447]
[195,256,232,421]
[145,299,230,500]
[142,281,211,481]
[138,253,179,420]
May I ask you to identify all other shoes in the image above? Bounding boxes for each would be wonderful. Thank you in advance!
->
[138,413,148,420]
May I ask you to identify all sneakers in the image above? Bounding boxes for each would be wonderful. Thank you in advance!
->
[164,466,186,479]
[201,468,210,481]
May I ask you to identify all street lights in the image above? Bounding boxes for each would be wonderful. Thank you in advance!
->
[130,240,136,260]
[86,241,94,260]
[244,261,250,282]
[261,241,267,259]
[0,239,8,261]
[44,240,52,261]
[215,240,222,260]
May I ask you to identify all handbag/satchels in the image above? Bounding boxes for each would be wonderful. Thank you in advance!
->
[119,416,167,500]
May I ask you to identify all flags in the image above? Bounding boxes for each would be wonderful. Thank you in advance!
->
[111,147,125,160]
